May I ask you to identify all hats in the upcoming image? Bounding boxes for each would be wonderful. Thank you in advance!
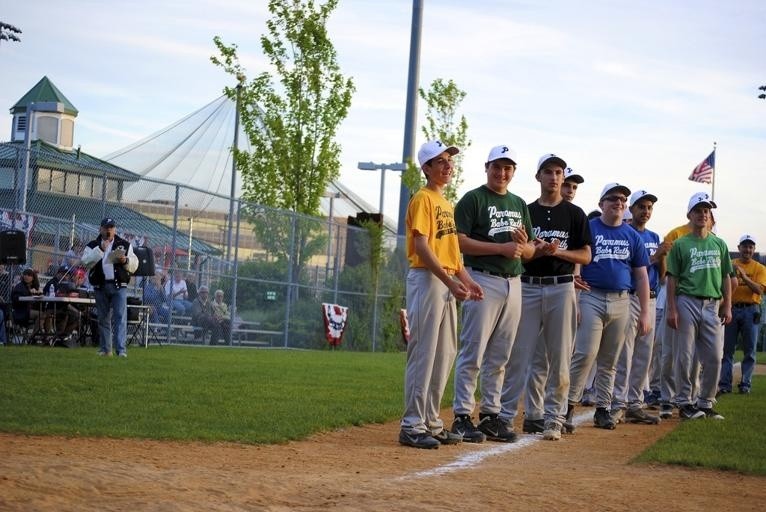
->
[101,218,117,227]
[58,265,87,278]
[623,208,634,220]
[487,144,519,168]
[687,191,716,214]
[536,154,585,184]
[627,189,656,208]
[600,182,632,198]
[417,140,460,168]
[738,233,754,245]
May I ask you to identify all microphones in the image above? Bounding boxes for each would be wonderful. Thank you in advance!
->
[107,231,110,242]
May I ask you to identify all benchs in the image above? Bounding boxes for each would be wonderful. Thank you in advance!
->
[0,276,285,347]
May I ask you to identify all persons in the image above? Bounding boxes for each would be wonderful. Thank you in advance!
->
[450,143,537,444]
[520,165,766,438]
[395,138,486,448]
[0,216,232,358]
[497,150,593,442]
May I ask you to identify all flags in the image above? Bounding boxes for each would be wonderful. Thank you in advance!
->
[688,149,716,184]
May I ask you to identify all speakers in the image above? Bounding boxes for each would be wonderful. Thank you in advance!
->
[0,230,26,264]
[130,247,155,276]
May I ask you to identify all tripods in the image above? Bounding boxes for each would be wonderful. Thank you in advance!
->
[0,264,21,345]
[128,277,162,346]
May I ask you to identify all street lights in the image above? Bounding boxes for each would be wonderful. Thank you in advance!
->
[358,163,408,350]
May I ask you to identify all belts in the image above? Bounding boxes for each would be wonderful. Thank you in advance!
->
[413,266,457,276]
[627,288,656,299]
[677,292,721,301]
[520,274,574,285]
[464,264,521,279]
[732,301,758,307]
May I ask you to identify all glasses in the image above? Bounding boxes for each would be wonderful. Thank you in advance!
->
[608,195,627,203]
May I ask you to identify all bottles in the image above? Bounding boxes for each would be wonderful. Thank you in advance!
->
[48,283,55,298]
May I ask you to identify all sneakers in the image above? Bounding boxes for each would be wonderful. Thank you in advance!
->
[29,331,76,346]
[737,384,752,396]
[398,403,576,449]
[582,384,732,430]
[98,348,128,359]
